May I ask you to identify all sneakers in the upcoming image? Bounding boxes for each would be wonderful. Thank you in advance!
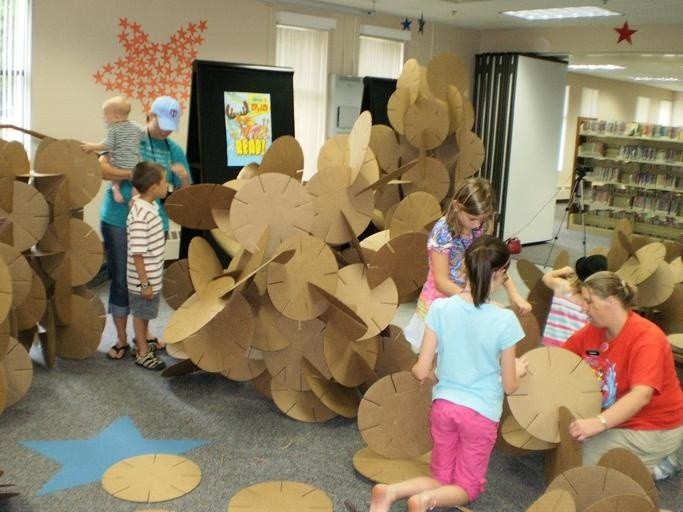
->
[130,338,166,370]
[660,440,683,476]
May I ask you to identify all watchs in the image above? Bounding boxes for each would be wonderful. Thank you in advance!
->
[135,280,151,290]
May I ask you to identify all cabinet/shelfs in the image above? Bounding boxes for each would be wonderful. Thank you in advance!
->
[568,116,683,242]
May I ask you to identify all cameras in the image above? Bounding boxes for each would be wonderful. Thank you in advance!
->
[575,163,594,178]
[160,183,174,203]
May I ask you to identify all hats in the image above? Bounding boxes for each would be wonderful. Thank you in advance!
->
[151,97,181,133]
[574,255,608,279]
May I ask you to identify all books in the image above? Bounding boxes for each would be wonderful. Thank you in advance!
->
[571,120,682,233]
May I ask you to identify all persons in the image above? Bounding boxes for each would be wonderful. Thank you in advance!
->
[366,233,529,511]
[559,268,682,485]
[401,174,534,356]
[76,94,146,204]
[97,95,194,363]
[122,161,169,373]
[536,251,609,350]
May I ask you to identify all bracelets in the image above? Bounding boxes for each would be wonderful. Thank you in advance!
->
[596,413,610,434]
[176,170,188,179]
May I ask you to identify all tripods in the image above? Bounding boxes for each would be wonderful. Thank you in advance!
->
[543,177,587,269]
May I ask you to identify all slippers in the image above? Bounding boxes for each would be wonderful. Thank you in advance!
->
[106,343,130,360]
